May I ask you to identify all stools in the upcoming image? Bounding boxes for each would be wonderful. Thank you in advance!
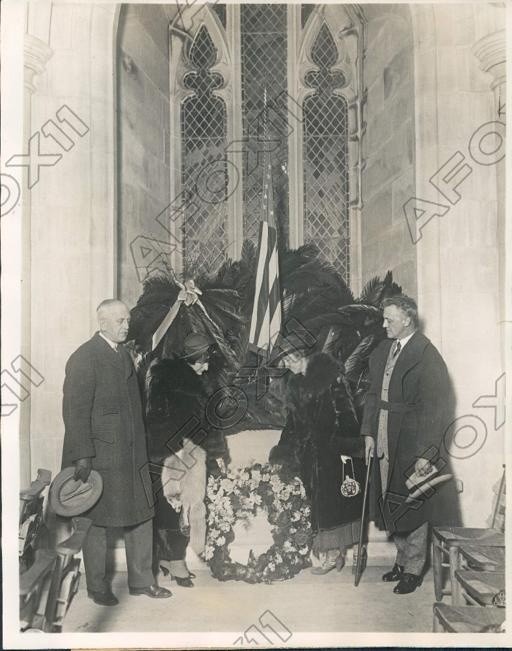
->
[425,461,506,633]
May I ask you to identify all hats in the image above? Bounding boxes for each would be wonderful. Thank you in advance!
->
[268,331,310,367]
[403,464,453,504]
[50,466,103,518]
[175,331,217,360]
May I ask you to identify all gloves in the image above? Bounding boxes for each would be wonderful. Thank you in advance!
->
[73,458,93,484]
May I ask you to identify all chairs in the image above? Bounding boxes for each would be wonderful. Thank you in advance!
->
[19,463,92,634]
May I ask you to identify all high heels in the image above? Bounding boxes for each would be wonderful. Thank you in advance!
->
[159,563,197,588]
[311,550,344,575]
[352,549,367,575]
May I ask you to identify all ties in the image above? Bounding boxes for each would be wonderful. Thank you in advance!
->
[392,343,402,359]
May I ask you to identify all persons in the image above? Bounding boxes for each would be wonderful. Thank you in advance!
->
[358,295,448,593]
[269,352,367,577]
[143,334,218,586]
[60,298,172,605]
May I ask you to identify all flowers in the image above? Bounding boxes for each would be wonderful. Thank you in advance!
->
[202,461,315,585]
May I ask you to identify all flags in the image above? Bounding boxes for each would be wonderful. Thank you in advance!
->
[247,152,283,367]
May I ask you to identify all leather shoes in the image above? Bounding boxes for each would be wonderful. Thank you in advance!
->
[87,588,118,606]
[129,585,173,599]
[383,564,404,582]
[393,573,424,594]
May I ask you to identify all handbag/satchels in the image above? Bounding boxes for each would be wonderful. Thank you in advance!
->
[340,457,361,498]
